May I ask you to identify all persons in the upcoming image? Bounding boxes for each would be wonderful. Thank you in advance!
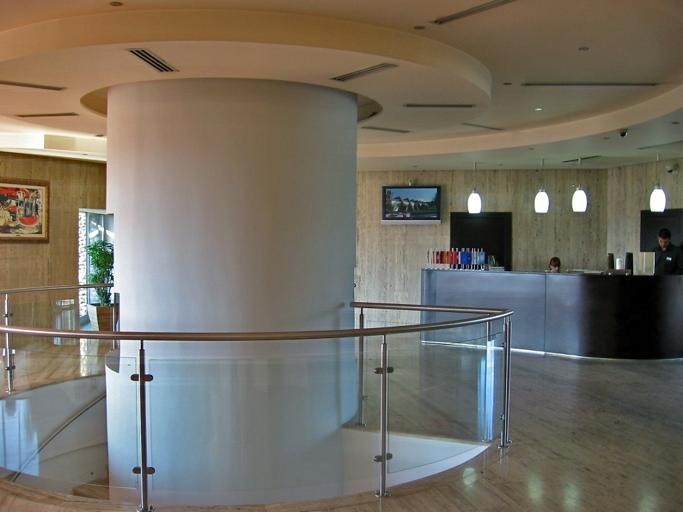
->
[652,228,683,275]
[549,257,560,273]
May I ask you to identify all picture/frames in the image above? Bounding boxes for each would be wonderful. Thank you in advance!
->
[1,176,50,244]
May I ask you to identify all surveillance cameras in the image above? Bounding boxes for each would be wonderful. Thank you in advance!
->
[620,131,628,139]
[665,162,678,174]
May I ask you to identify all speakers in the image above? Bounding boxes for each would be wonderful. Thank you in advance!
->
[608,253,614,275]
[624,252,633,275]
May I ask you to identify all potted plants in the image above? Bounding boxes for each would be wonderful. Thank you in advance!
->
[82,239,120,331]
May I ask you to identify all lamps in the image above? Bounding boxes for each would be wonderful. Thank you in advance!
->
[466,152,667,215]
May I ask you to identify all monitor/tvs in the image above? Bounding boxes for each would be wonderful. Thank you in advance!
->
[380,185,441,225]
